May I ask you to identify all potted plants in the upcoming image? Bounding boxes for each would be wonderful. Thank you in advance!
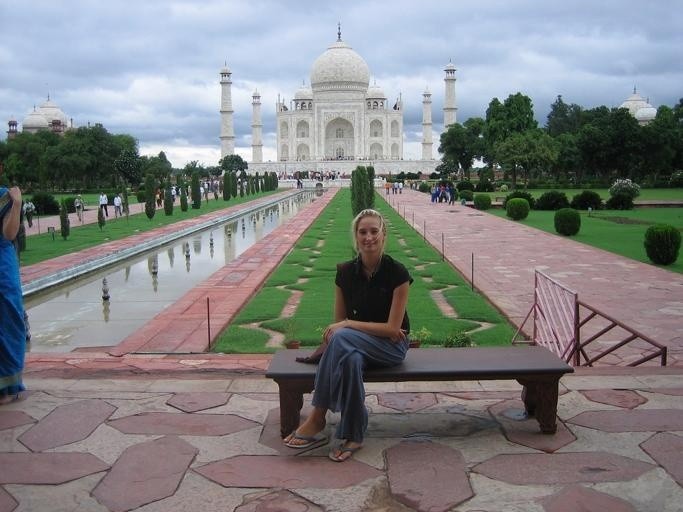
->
[409,329,432,347]
[281,334,300,350]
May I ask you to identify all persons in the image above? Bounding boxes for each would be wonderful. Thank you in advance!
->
[155,178,247,209]
[276,168,345,191]
[428,182,455,206]
[0,185,29,408]
[383,180,403,195]
[98,191,108,217]
[409,178,414,189]
[113,193,122,219]
[22,198,36,228]
[73,194,84,222]
[278,206,413,464]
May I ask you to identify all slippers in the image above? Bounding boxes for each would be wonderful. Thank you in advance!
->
[329,437,363,461]
[283,428,327,449]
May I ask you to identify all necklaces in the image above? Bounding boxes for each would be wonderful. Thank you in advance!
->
[361,256,380,279]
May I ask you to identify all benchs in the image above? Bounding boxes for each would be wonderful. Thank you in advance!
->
[264,343,574,440]
